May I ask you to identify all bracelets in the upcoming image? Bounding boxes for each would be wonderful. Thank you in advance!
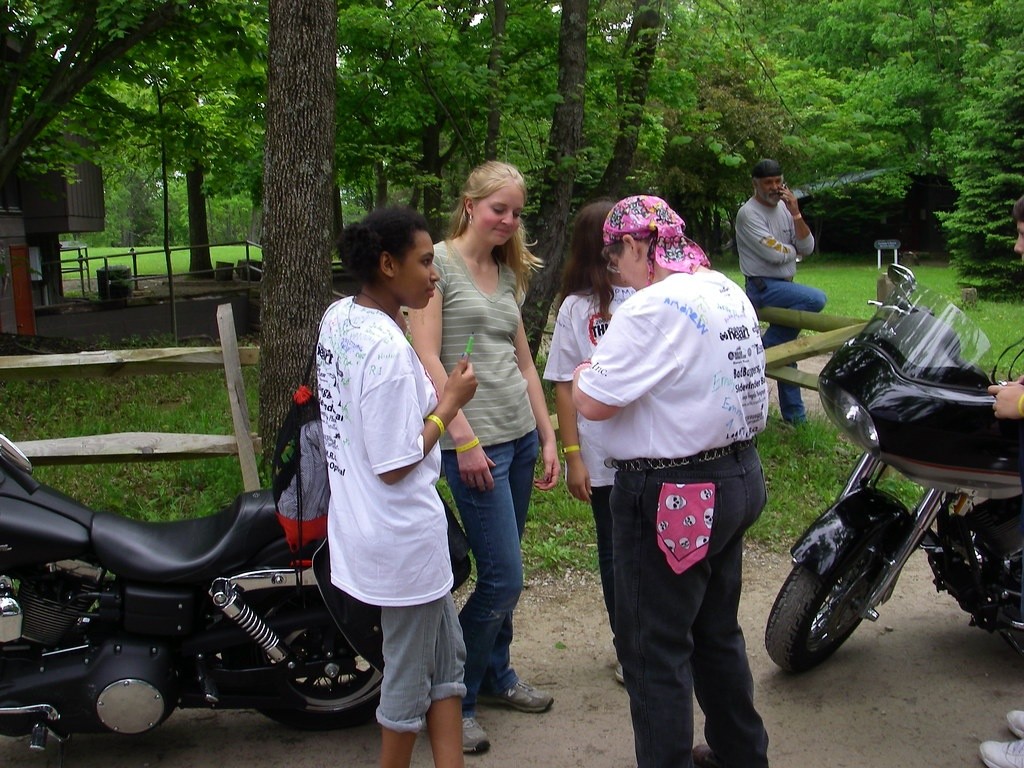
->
[456,436,480,453]
[1017,395,1024,417]
[793,212,802,220]
[573,360,591,378]
[424,414,444,441]
[560,444,580,455]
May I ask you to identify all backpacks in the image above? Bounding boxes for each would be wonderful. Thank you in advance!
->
[272,305,340,584]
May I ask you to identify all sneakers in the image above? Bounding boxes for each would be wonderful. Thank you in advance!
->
[473,681,554,713]
[458,717,491,753]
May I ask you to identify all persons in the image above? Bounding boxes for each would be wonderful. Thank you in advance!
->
[736,159,826,433]
[313,206,480,768]
[975,192,1024,768]
[406,160,560,755]
[571,194,773,768]
[542,198,641,681]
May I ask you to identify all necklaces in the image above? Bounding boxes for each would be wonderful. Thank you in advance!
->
[360,291,397,323]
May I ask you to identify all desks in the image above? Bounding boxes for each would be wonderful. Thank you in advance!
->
[60,240,91,298]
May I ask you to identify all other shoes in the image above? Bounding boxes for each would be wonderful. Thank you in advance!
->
[616,663,623,682]
[979,739,1024,768]
[692,744,768,768]
[1007,710,1024,738]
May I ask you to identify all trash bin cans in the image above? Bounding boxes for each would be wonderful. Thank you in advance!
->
[96,265,134,298]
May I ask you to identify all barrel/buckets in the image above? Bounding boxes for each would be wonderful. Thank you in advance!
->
[215,261,234,281]
[96,265,132,300]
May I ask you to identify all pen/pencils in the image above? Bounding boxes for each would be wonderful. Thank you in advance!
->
[465,330,474,357]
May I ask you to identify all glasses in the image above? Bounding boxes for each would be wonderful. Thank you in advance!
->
[607,243,625,273]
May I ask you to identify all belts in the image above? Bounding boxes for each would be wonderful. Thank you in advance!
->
[745,275,793,282]
[615,438,755,471]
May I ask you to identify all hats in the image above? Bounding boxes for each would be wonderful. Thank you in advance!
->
[751,159,783,179]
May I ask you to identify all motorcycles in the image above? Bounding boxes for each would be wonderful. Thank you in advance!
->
[0,339,384,742]
[762,260,1024,677]
